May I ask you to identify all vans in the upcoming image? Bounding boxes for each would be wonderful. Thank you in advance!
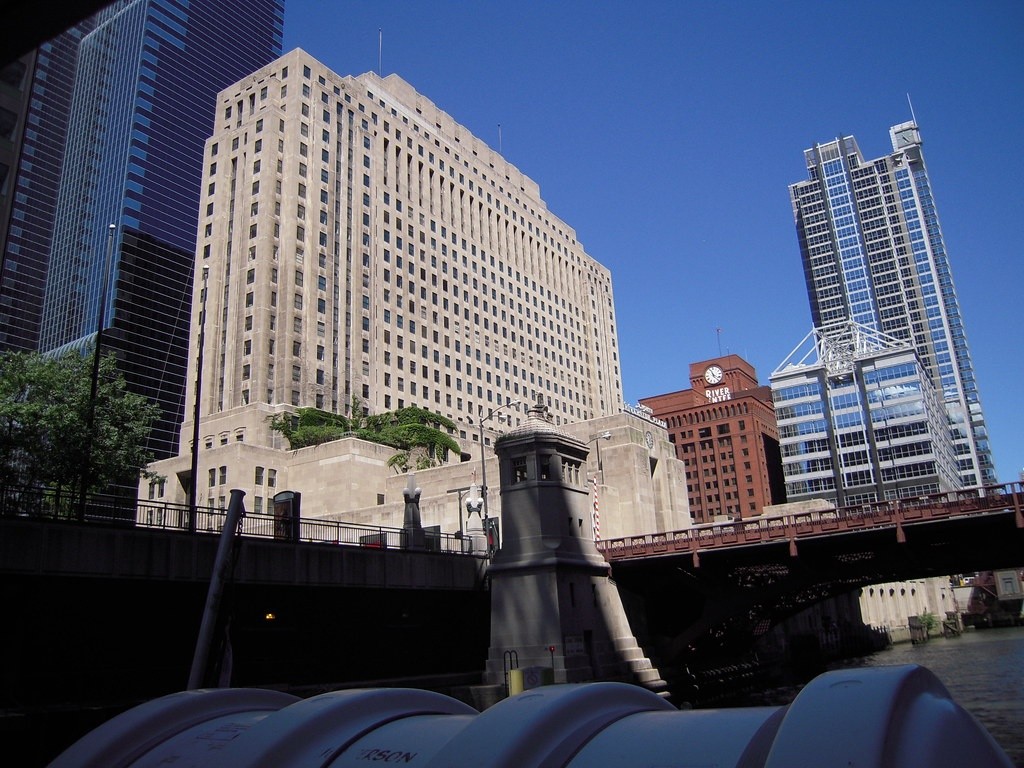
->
[964,577,974,584]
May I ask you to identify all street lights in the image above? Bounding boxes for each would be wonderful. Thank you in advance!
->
[188,265,210,531]
[79,224,116,520]
[480,400,521,553]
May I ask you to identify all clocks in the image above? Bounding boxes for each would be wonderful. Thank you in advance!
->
[894,129,915,148]
[704,364,723,385]
